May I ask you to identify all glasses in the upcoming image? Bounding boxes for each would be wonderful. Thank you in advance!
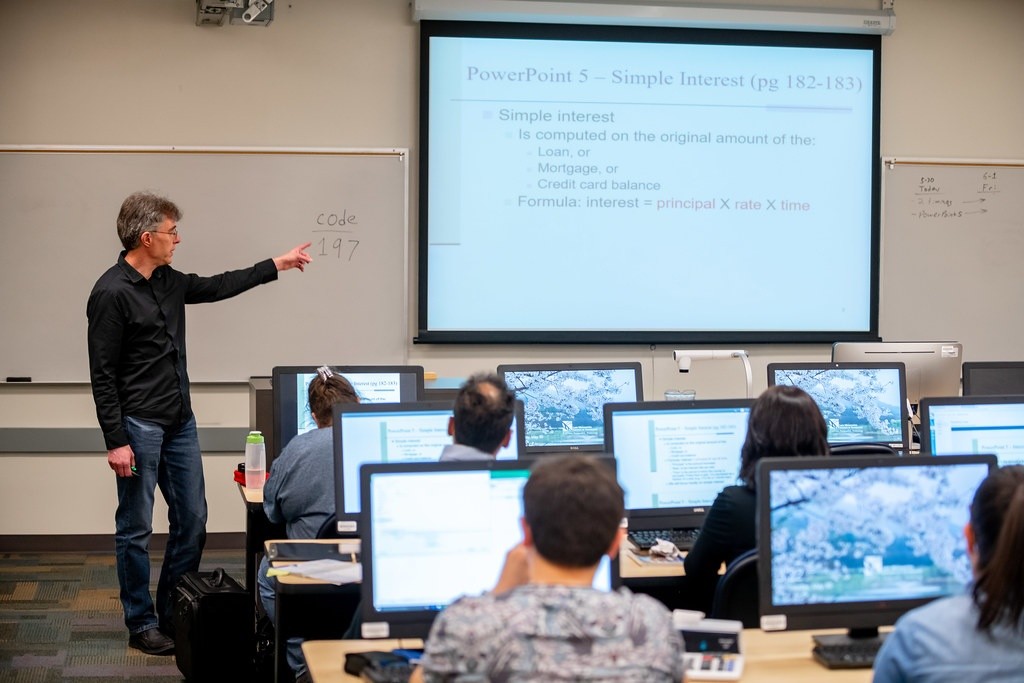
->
[149,229,178,240]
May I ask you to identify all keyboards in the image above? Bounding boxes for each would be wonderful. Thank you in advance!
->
[363,663,417,683]
[627,529,702,551]
[812,641,884,669]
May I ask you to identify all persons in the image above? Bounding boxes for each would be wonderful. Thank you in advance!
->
[683,385,829,620]
[86,192,312,655]
[258,366,361,683]
[438,371,516,461]
[871,463,1024,683]
[411,452,685,683]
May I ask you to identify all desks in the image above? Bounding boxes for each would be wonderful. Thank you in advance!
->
[237,477,893,683]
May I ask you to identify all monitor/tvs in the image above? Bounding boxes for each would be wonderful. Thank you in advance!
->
[273,342,1023,644]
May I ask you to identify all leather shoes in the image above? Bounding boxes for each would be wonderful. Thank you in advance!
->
[129,629,174,654]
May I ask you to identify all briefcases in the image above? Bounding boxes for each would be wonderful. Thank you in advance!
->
[175,567,255,683]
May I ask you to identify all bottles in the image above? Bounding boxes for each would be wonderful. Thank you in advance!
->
[244,427,267,491]
[423,371,437,389]
[664,389,696,401]
